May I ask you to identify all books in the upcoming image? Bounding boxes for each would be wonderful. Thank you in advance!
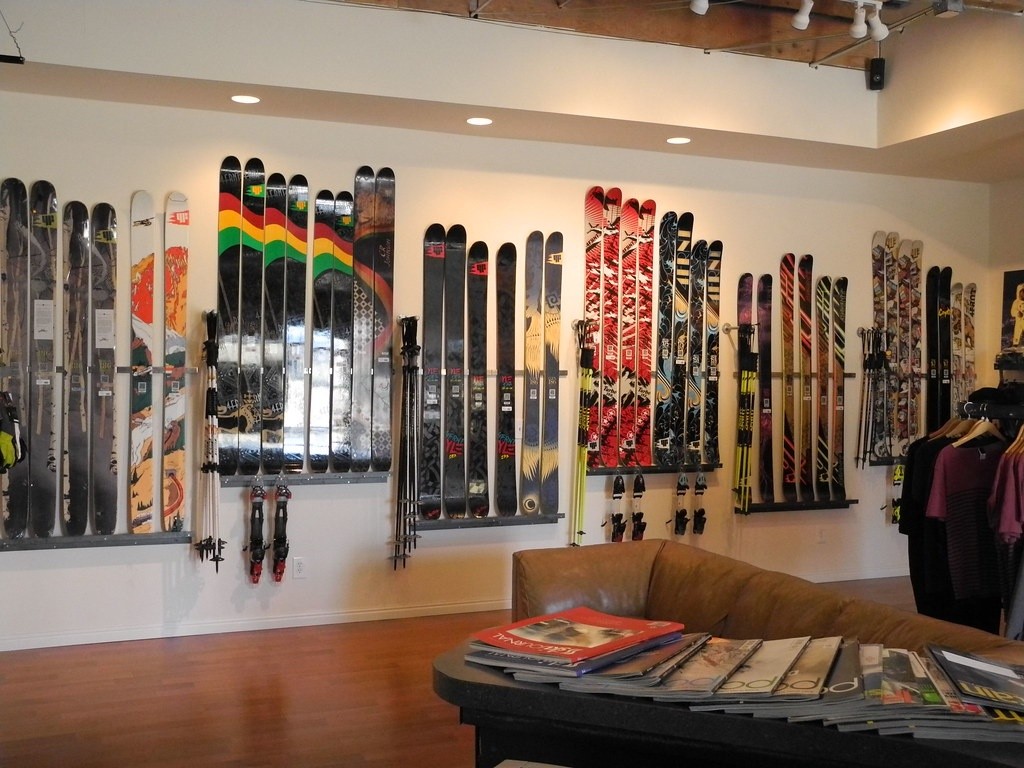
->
[466,605,1024,741]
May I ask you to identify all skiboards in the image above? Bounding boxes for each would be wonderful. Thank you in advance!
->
[0,154,979,542]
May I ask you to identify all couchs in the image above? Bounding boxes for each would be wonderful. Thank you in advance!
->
[511,537,1024,671]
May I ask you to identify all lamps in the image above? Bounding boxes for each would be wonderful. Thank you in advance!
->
[868,0,889,42]
[688,0,709,15]
[790,1,814,30]
[849,1,867,39]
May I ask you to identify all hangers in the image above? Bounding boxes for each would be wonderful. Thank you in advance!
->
[927,400,1024,455]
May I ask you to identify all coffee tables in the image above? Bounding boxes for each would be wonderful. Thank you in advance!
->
[428,648,1024,768]
